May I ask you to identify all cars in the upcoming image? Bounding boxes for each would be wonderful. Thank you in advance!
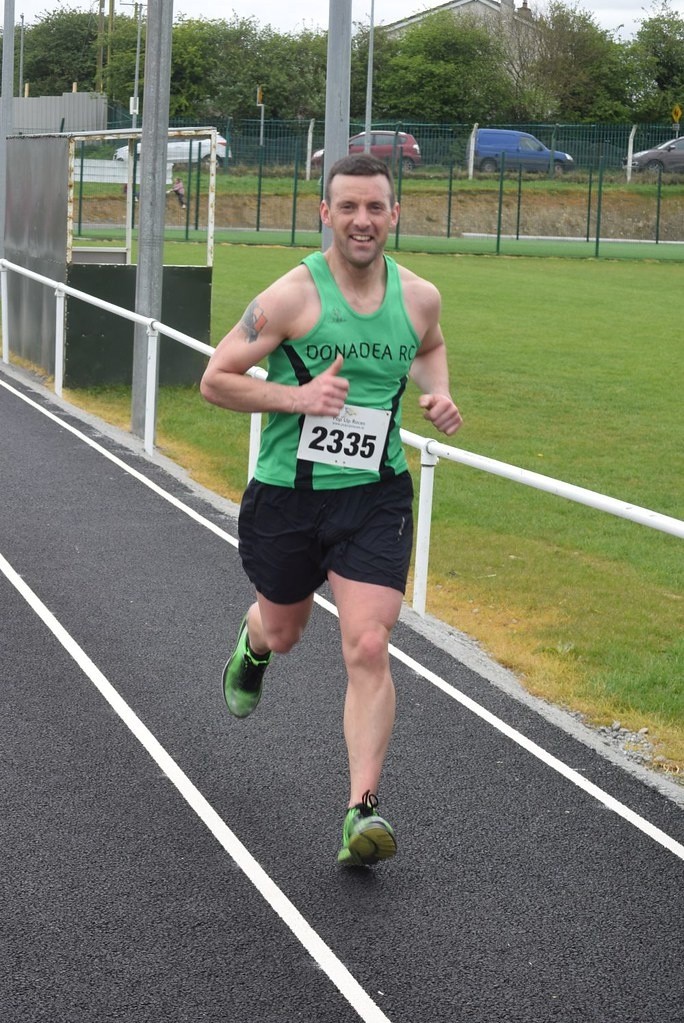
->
[621,135,684,176]
[311,129,422,173]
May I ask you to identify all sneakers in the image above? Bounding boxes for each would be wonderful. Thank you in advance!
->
[337,789,398,868]
[221,615,274,718]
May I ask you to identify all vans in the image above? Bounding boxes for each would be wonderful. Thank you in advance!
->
[474,129,574,173]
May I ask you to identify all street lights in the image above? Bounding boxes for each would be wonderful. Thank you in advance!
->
[18,13,24,96]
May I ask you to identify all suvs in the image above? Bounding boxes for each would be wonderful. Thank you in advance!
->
[113,128,234,172]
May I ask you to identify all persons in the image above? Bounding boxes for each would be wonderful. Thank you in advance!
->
[201,155,462,864]
[169,178,186,208]
